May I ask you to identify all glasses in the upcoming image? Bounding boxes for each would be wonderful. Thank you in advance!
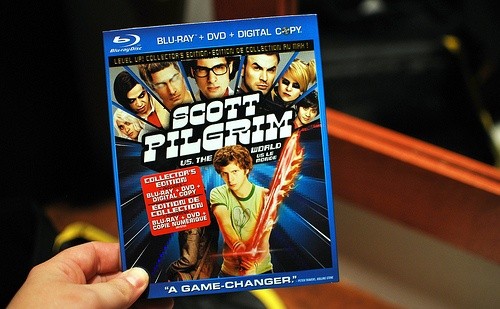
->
[191,62,228,78]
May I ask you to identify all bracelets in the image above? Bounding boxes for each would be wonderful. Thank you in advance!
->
[232,240,242,255]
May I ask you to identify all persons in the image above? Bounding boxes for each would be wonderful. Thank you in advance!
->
[237,52,281,96]
[208,144,275,279]
[268,59,315,108]
[5,243,174,308]
[289,91,318,130]
[138,62,193,106]
[112,108,145,144]
[114,71,169,130]
[196,58,234,99]
